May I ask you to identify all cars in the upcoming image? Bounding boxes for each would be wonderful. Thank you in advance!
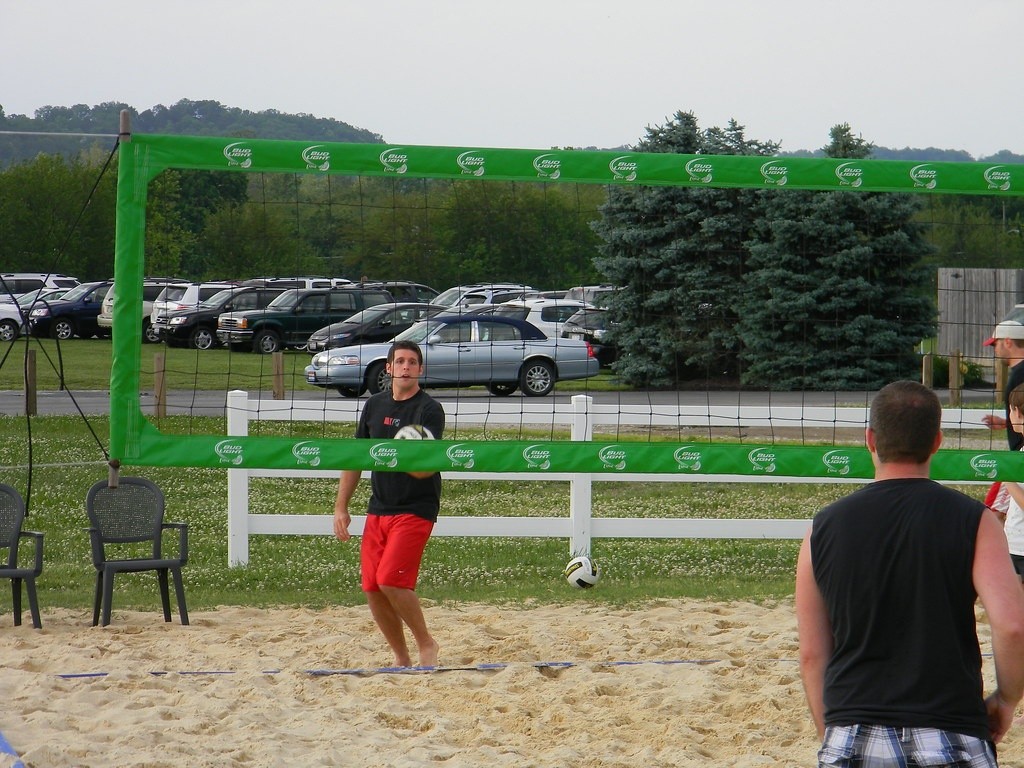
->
[306,302,449,356]
[0,287,78,342]
[303,315,600,399]
[412,304,500,331]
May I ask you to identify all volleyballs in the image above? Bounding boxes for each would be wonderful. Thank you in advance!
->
[392,425,434,440]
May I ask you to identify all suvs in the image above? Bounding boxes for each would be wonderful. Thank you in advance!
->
[240,278,625,305]
[216,285,402,354]
[152,284,296,350]
[20,281,113,340]
[0,273,82,304]
[150,281,238,325]
[96,275,177,343]
[560,307,630,365]
[492,298,596,338]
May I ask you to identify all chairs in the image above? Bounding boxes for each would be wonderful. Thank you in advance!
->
[0,483,44,629]
[81,477,190,628]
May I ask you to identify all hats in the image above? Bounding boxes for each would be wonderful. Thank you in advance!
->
[981,320,1024,347]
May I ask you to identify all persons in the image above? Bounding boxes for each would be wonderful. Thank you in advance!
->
[796,381,1024,768]
[333,341,445,666]
[1003,383,1024,587]
[981,320,1024,525]
